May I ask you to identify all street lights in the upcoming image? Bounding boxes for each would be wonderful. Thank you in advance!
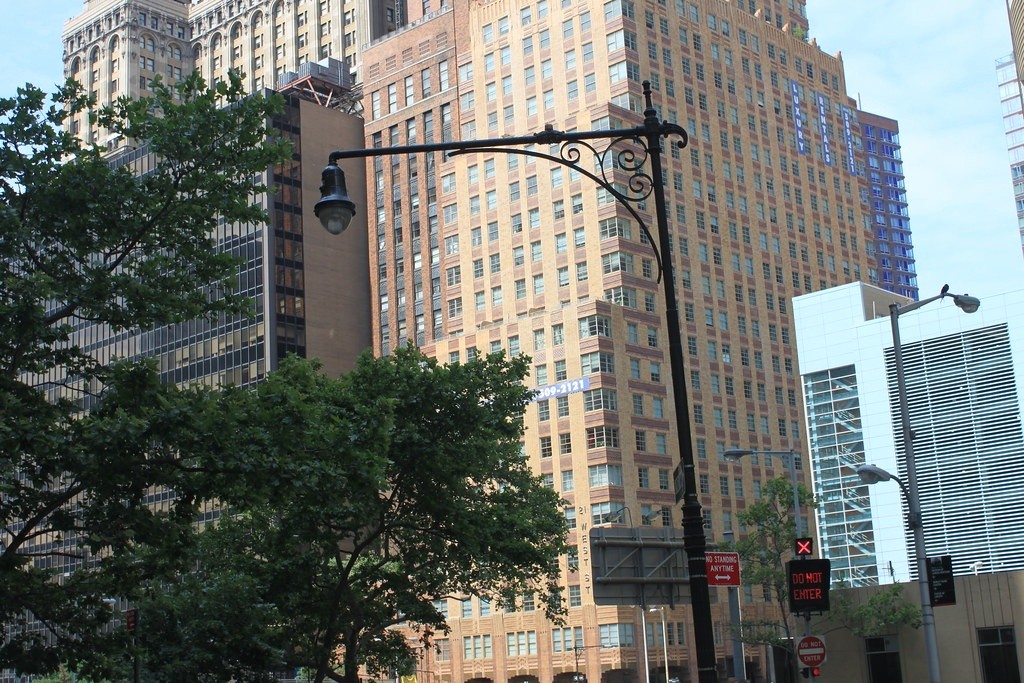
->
[307,79,724,683]
[721,448,820,683]
[856,284,985,683]
[603,504,652,683]
[648,508,692,683]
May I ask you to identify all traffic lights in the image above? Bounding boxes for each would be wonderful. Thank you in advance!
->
[800,667,809,679]
[811,668,820,676]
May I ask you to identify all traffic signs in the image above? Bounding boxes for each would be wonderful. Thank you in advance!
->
[705,551,742,589]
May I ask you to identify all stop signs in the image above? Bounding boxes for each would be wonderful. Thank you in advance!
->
[797,636,825,667]
